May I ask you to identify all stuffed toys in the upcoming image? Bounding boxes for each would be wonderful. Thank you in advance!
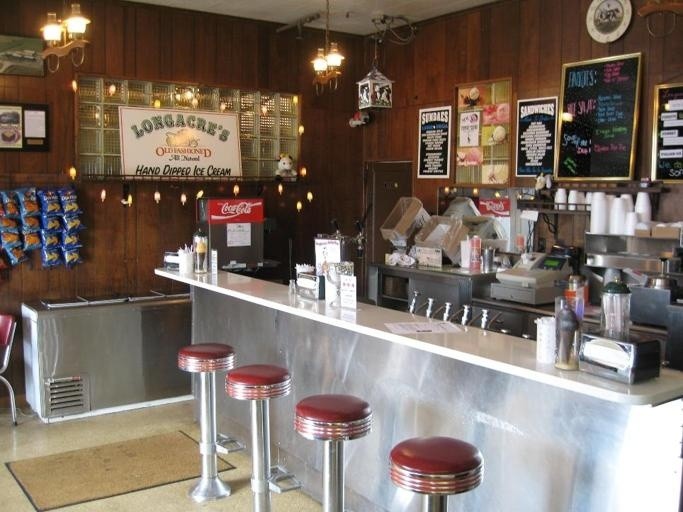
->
[275,153,298,182]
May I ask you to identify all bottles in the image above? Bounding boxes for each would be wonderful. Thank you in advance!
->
[193,232,208,273]
[600,283,632,337]
[288,279,296,294]
[470,235,481,271]
[569,275,584,291]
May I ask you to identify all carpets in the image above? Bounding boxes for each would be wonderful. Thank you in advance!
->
[3,428,237,511]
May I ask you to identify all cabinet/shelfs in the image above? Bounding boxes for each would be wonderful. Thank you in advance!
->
[377,270,470,323]
[471,307,536,342]
[70,73,301,187]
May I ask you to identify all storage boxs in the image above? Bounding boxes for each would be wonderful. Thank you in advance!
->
[378,196,471,269]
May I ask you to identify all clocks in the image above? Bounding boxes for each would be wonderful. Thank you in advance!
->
[585,0,631,44]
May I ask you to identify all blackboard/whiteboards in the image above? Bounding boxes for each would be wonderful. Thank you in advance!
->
[553,52,644,182]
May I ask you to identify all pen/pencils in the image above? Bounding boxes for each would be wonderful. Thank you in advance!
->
[178,243,194,252]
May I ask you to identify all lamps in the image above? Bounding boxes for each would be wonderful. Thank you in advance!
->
[309,1,346,97]
[37,1,92,74]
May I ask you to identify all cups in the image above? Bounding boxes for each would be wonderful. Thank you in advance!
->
[179,252,193,272]
[555,296,584,370]
[534,317,556,365]
[554,188,652,237]
[483,247,494,271]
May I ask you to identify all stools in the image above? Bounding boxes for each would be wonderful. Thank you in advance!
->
[179,344,247,503]
[388,437,485,511]
[295,396,373,511]
[226,366,299,510]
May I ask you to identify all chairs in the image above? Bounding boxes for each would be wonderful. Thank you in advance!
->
[0,313,20,426]
